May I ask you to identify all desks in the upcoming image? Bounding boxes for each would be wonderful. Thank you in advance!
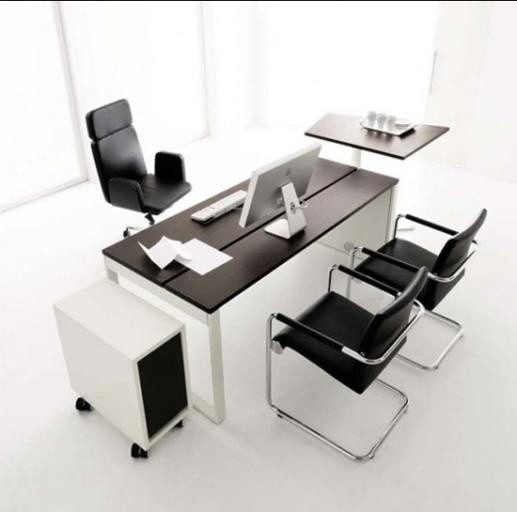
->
[102,159,400,425]
[304,112,450,171]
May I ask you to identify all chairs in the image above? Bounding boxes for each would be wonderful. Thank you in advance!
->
[265,265,430,462]
[85,100,191,237]
[345,207,488,371]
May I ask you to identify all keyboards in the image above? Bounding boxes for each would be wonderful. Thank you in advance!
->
[191,190,248,225]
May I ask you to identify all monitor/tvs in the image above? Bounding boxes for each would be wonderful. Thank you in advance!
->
[238,142,322,240]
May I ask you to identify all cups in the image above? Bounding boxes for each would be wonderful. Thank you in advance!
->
[366,110,396,132]
